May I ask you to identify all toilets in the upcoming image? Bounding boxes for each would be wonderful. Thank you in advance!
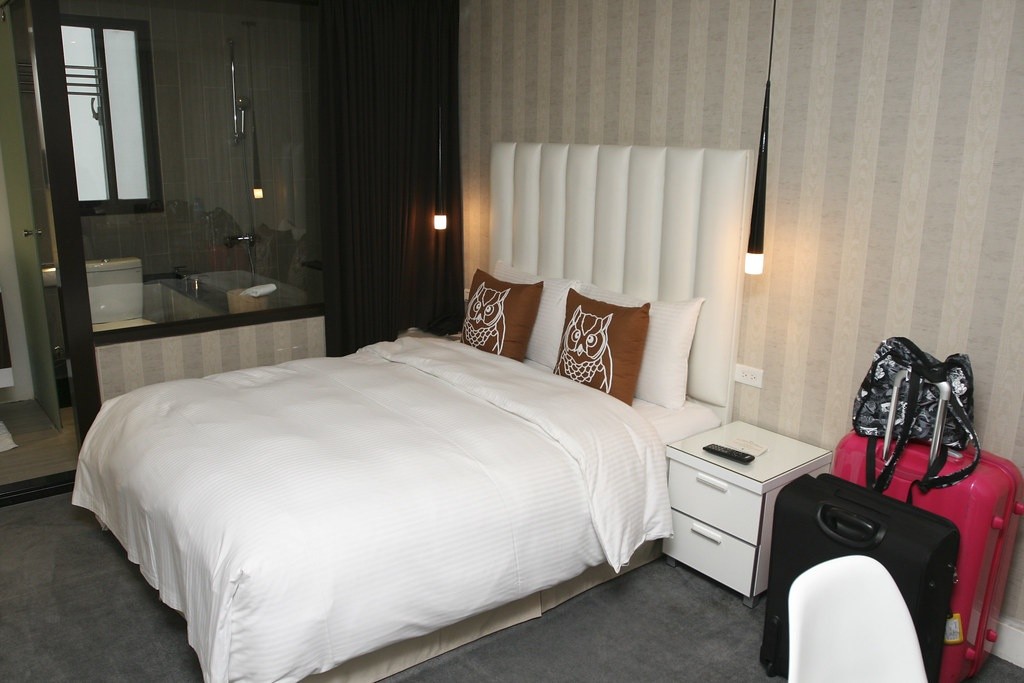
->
[84,255,160,336]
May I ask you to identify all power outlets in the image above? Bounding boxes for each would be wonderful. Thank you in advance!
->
[733,364,764,390]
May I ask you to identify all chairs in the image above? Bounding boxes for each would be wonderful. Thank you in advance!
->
[784,554,934,683]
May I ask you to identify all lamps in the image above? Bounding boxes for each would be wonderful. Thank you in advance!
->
[742,0,778,277]
[244,21,264,200]
[432,0,450,231]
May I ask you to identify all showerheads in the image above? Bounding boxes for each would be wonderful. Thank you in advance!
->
[237,94,250,136]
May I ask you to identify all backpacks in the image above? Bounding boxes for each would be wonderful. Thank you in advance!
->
[853,337,983,498]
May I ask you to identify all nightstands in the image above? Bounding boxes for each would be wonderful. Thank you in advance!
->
[659,420,836,611]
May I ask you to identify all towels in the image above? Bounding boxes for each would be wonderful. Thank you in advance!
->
[239,282,276,300]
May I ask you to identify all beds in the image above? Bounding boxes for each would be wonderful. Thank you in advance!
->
[68,330,733,683]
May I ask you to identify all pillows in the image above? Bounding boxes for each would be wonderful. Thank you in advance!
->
[487,257,582,370]
[553,287,650,408]
[576,281,706,412]
[459,269,545,363]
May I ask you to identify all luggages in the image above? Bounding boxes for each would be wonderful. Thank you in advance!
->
[830,428,1024,683]
[757,472,960,683]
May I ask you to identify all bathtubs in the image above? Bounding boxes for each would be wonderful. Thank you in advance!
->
[193,268,310,311]
